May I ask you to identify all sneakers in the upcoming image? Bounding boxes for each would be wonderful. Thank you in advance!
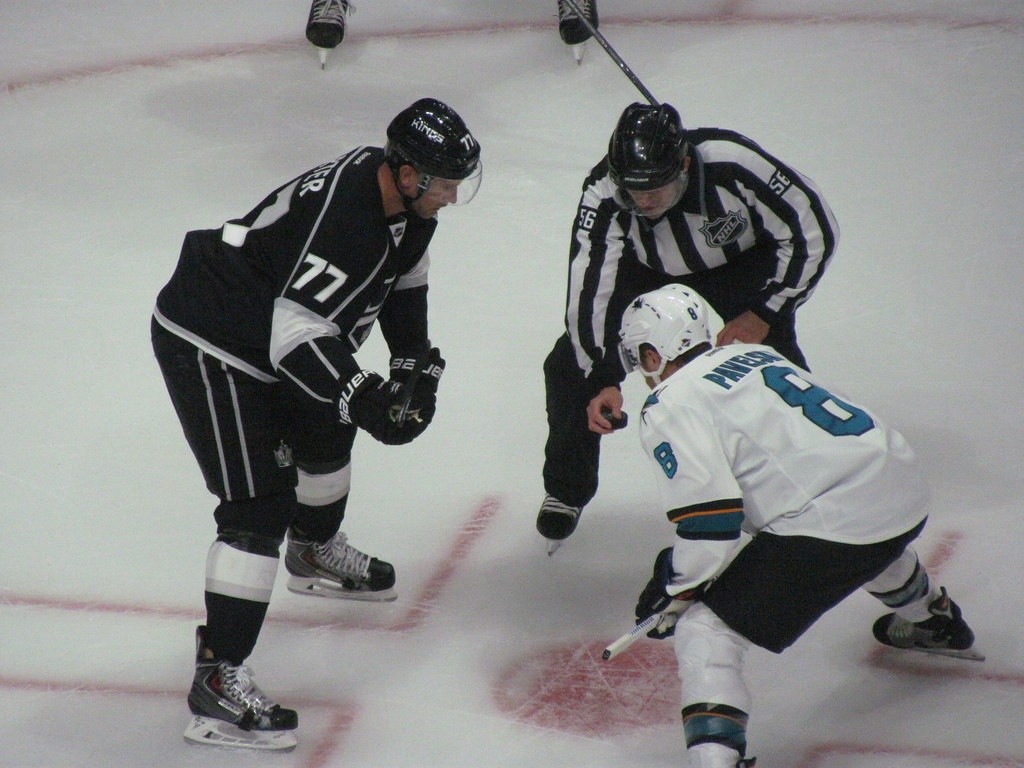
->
[285,523,398,600]
[305,0,356,69]
[535,490,584,555]
[736,756,757,768]
[183,625,298,749]
[557,0,599,65]
[872,585,986,661]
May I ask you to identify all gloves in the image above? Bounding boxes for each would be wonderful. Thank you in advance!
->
[388,347,446,424]
[635,547,709,639]
[331,366,436,445]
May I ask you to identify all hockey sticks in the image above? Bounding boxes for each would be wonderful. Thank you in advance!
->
[392,335,433,429]
[602,534,756,663]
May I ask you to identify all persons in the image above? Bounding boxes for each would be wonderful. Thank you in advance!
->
[305,0,599,70]
[149,97,483,752]
[616,284,988,768]
[537,104,842,554]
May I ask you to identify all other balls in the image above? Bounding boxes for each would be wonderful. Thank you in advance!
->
[602,409,629,430]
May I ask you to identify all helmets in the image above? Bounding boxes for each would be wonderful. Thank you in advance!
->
[606,102,687,190]
[616,284,711,377]
[386,97,481,180]
